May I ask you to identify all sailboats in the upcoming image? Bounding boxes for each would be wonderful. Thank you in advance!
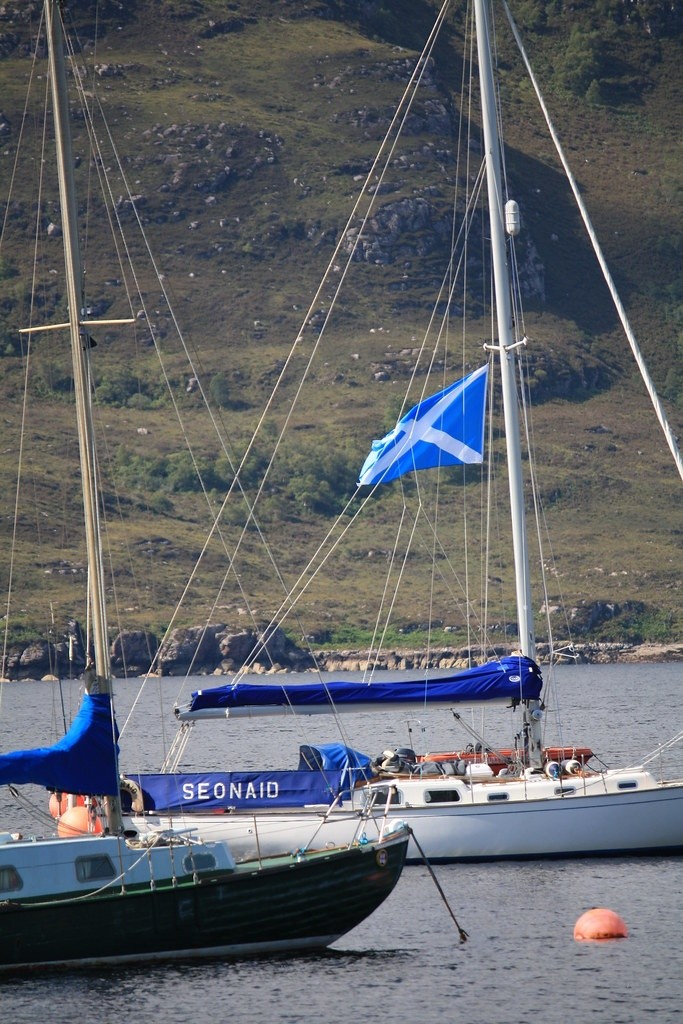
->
[2,0,413,974]
[52,0,683,859]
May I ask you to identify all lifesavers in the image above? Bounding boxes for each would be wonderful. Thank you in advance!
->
[105,780,144,817]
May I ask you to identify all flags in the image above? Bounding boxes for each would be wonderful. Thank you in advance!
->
[355,362,489,487]
